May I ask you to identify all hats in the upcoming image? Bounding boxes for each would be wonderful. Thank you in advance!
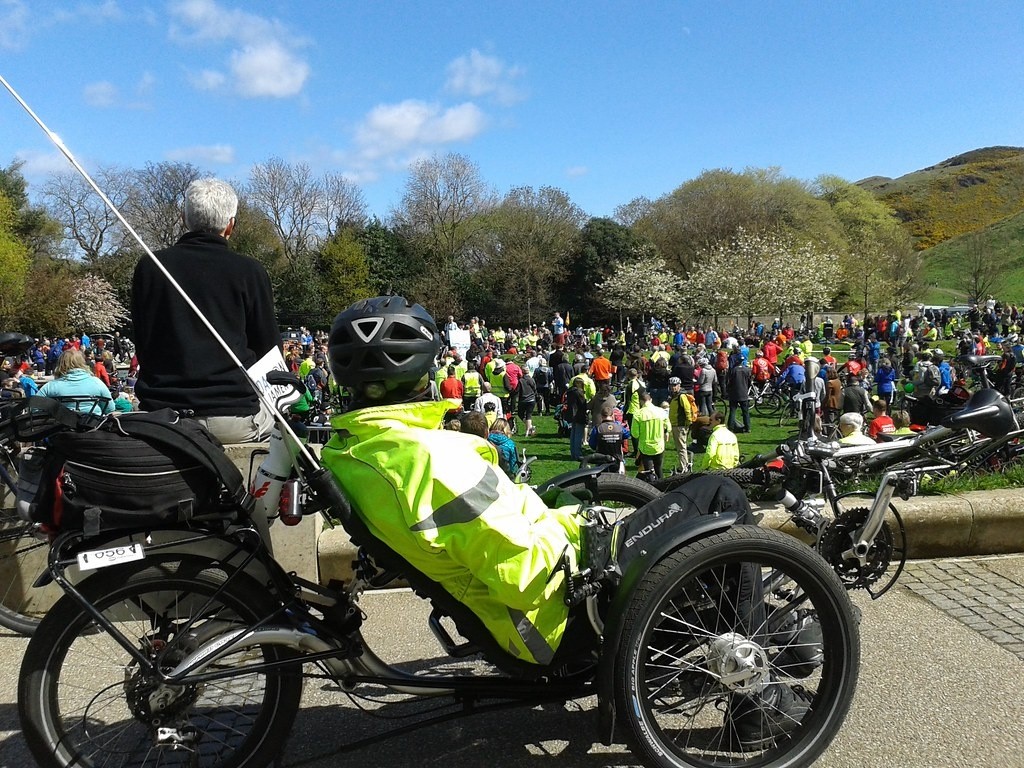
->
[848,354,856,359]
[756,350,763,357]
[539,358,547,366]
[659,344,665,350]
[681,346,687,354]
[495,361,504,368]
[794,348,802,353]
[699,358,709,365]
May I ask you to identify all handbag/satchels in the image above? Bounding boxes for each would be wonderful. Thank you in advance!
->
[633,379,646,409]
[10,396,275,559]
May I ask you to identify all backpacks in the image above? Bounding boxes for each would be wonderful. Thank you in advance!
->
[925,364,941,387]
[678,393,698,421]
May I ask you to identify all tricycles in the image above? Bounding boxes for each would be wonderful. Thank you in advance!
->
[17,371,921,768]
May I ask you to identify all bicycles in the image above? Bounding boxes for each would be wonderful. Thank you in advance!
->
[653,355,1024,498]
[0,388,111,638]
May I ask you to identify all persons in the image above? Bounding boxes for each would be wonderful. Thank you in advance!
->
[320,296,863,751]
[0,332,140,447]
[130,179,341,444]
[786,297,1024,496]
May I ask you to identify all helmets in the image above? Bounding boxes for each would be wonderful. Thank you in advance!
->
[327,296,439,386]
[668,377,681,384]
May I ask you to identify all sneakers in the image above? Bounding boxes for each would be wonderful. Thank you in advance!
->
[715,675,817,752]
[783,606,861,670]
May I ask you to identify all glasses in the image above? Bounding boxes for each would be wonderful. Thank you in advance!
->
[839,423,851,428]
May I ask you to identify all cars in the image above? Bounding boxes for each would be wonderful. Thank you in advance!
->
[90,334,115,343]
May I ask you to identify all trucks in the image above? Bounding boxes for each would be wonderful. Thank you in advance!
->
[917,306,971,321]
